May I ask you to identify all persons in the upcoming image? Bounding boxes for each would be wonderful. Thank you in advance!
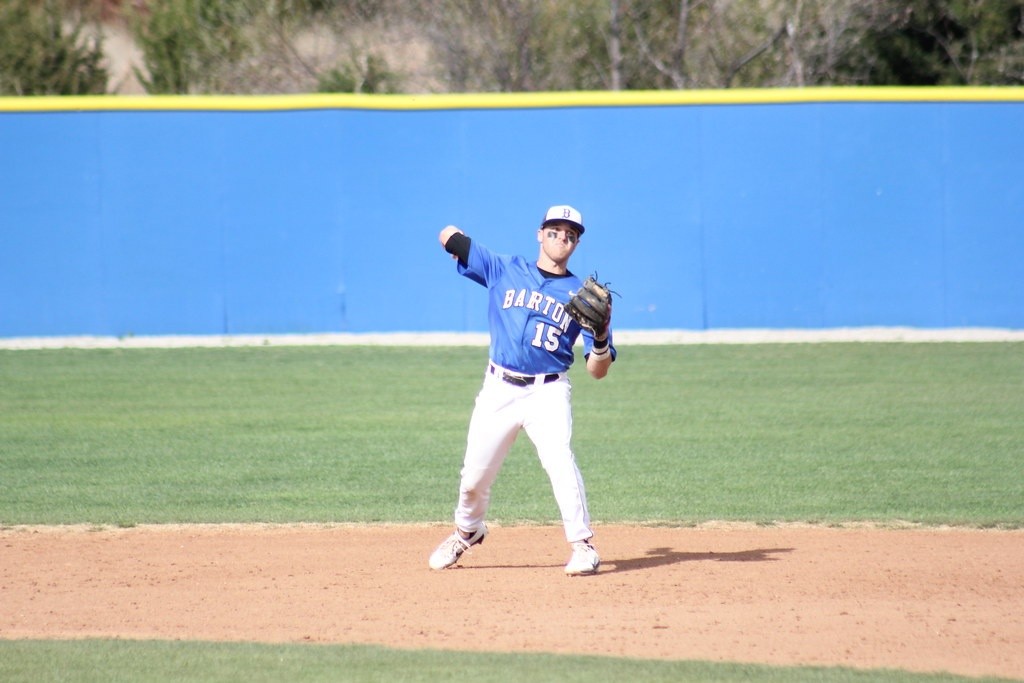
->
[430,204,616,575]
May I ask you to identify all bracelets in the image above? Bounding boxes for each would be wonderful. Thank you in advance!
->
[590,345,610,361]
[593,337,608,349]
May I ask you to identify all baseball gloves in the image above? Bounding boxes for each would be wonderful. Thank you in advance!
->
[562,269,623,338]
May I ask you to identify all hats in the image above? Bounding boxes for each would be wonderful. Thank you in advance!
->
[541,205,585,238]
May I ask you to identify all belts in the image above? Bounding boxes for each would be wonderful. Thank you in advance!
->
[491,364,558,388]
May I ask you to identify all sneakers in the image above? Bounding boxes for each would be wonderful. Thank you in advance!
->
[565,544,601,577]
[429,529,484,570]
